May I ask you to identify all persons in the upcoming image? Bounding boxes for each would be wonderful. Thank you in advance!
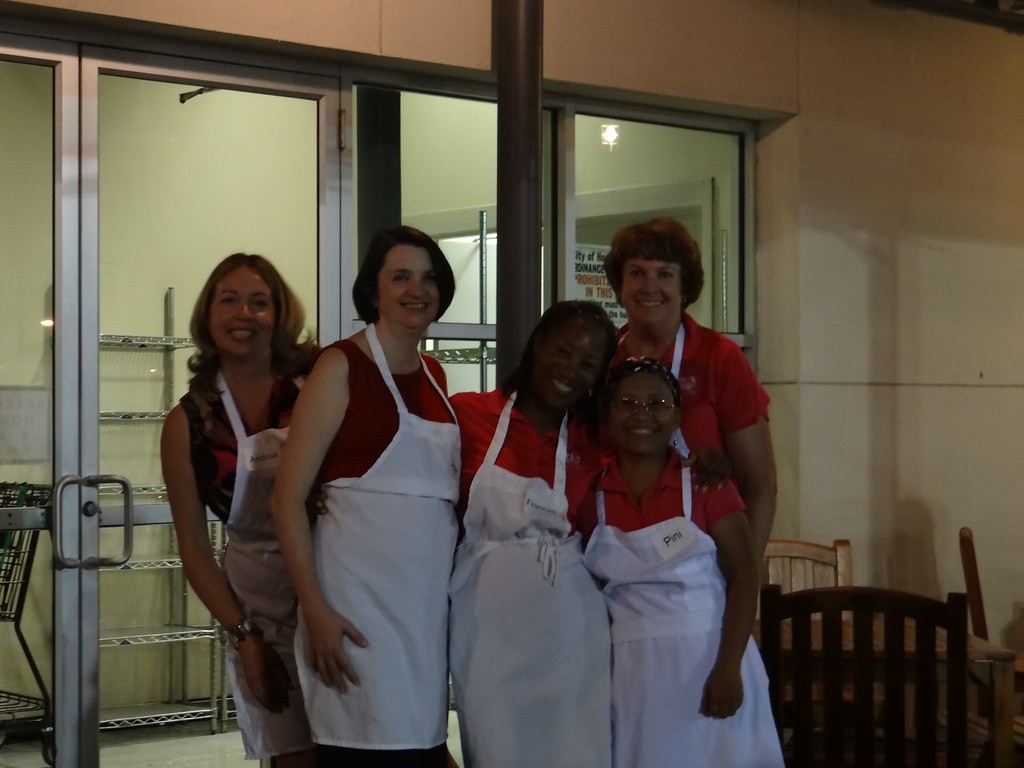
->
[305,300,732,768]
[268,224,465,768]
[588,217,778,566]
[577,358,786,768]
[160,254,327,768]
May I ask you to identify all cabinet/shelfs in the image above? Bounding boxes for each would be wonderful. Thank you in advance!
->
[99,287,218,743]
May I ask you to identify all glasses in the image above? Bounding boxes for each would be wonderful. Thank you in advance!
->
[609,391,676,419]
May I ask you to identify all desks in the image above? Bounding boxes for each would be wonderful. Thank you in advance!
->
[751,618,1017,768]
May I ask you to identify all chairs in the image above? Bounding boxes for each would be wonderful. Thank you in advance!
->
[760,583,972,768]
[765,537,854,621]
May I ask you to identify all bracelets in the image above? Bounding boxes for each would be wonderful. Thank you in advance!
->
[227,620,264,649]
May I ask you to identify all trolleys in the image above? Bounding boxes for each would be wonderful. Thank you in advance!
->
[1,482,58,761]
